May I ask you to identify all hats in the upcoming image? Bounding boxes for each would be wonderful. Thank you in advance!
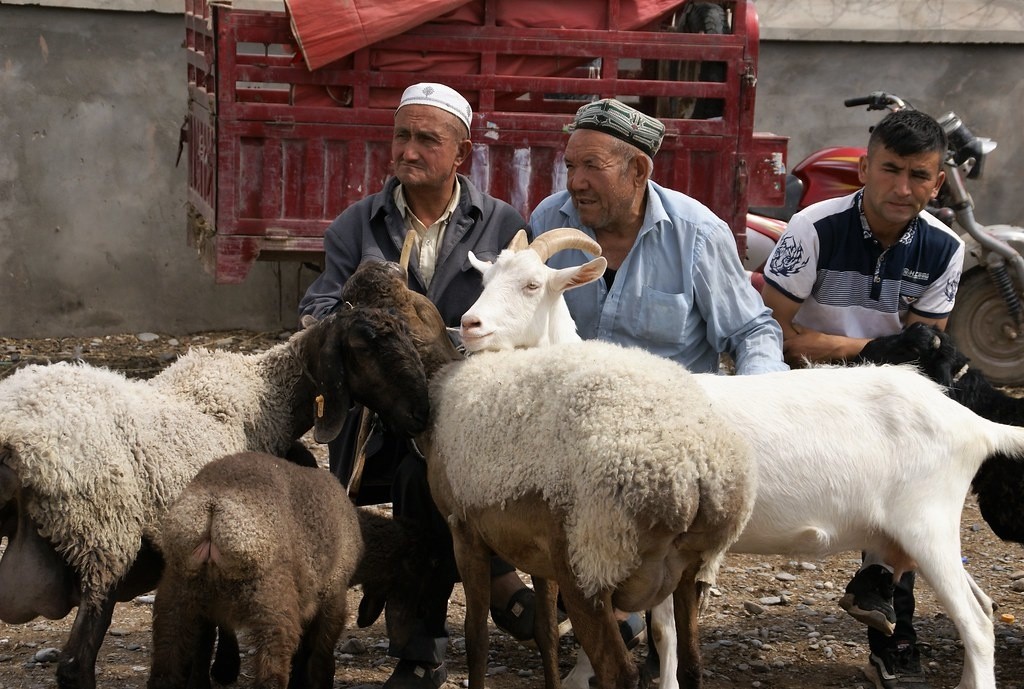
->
[393,81,475,140]
[573,98,667,158]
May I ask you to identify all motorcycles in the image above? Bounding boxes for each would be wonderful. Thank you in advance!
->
[171,0,1023,389]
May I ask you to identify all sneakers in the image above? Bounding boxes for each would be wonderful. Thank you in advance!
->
[490,585,573,650]
[866,627,927,689]
[380,662,448,688]
[353,583,388,630]
[838,563,903,637]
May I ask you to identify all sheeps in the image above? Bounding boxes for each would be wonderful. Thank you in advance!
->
[1,228,1024,689]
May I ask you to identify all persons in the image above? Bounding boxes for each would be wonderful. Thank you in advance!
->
[298,81,550,642]
[524,97,785,656]
[761,110,966,687]
